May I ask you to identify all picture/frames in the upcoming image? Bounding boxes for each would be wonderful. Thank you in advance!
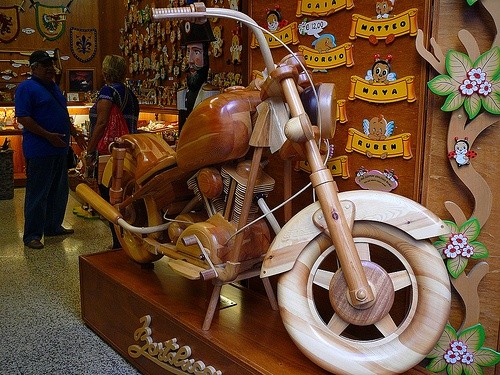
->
[66,67,97,92]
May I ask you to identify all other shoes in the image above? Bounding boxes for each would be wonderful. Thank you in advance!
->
[60,226,73,233]
[24,238,44,248]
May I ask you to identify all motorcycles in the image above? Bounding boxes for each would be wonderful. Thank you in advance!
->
[76,1,452,375]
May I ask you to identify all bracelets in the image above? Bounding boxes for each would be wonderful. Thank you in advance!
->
[73,132,80,137]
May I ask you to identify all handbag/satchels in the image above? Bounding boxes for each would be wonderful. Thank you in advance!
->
[96,87,130,155]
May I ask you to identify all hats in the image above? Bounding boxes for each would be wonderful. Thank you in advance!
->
[30,51,57,66]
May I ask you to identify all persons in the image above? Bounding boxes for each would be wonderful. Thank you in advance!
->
[175,21,214,137]
[16,50,90,248]
[89,55,139,249]
